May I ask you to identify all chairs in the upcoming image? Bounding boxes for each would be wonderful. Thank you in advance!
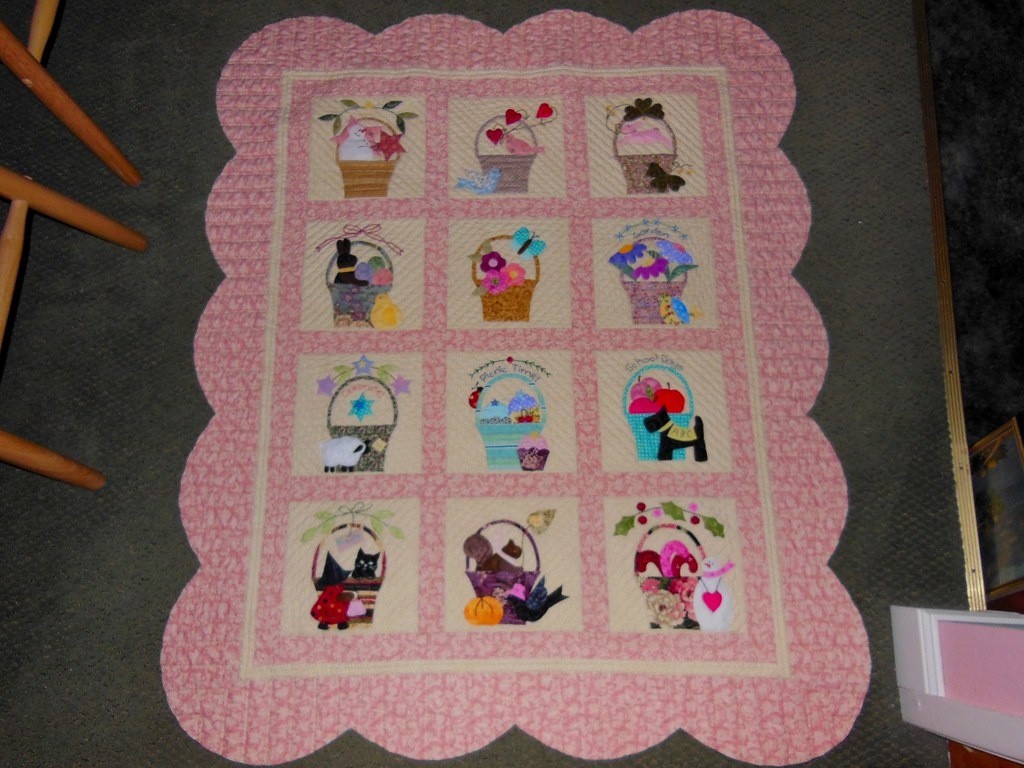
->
[0,0,148,489]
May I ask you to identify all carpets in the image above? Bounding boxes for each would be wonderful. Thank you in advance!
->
[158,5,870,768]
